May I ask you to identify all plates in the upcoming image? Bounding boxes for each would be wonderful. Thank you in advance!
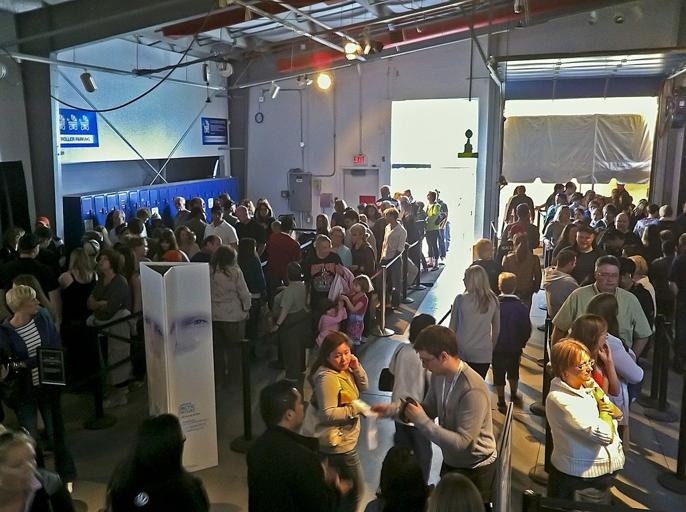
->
[63,177,239,251]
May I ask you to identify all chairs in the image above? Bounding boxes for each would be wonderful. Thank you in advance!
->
[431,266,439,271]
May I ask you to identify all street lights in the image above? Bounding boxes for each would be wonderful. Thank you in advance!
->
[379,368,394,391]
[311,391,352,408]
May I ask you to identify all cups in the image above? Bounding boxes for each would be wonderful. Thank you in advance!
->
[419,356,437,362]
[575,359,595,370]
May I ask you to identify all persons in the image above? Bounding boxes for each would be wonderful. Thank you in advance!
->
[547,314,620,397]
[105,414,210,511]
[502,232,542,313]
[491,272,532,409]
[550,255,654,364]
[389,313,436,496]
[534,182,685,374]
[308,333,369,510]
[317,297,348,349]
[505,185,535,223]
[498,204,540,259]
[588,292,643,445]
[428,473,485,510]
[341,276,369,352]
[547,337,625,505]
[425,189,450,271]
[1,193,300,407]
[2,427,74,510]
[247,382,353,510]
[370,325,498,510]
[471,239,503,297]
[300,186,428,348]
[365,445,430,510]
[269,262,311,383]
[12,275,60,343]
[449,265,500,380]
[2,286,77,483]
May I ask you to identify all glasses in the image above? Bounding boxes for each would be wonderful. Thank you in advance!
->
[295,76,304,87]
[268,82,280,100]
[80,69,98,92]
[303,74,314,86]
